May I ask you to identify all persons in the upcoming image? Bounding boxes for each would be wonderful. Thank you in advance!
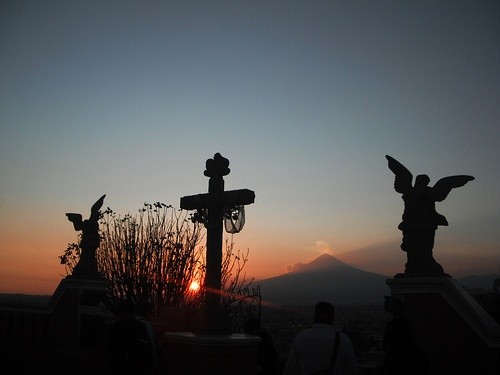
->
[398,173,449,273]
[283,300,360,375]
[258,331,278,374]
[74,218,102,273]
[243,317,261,337]
